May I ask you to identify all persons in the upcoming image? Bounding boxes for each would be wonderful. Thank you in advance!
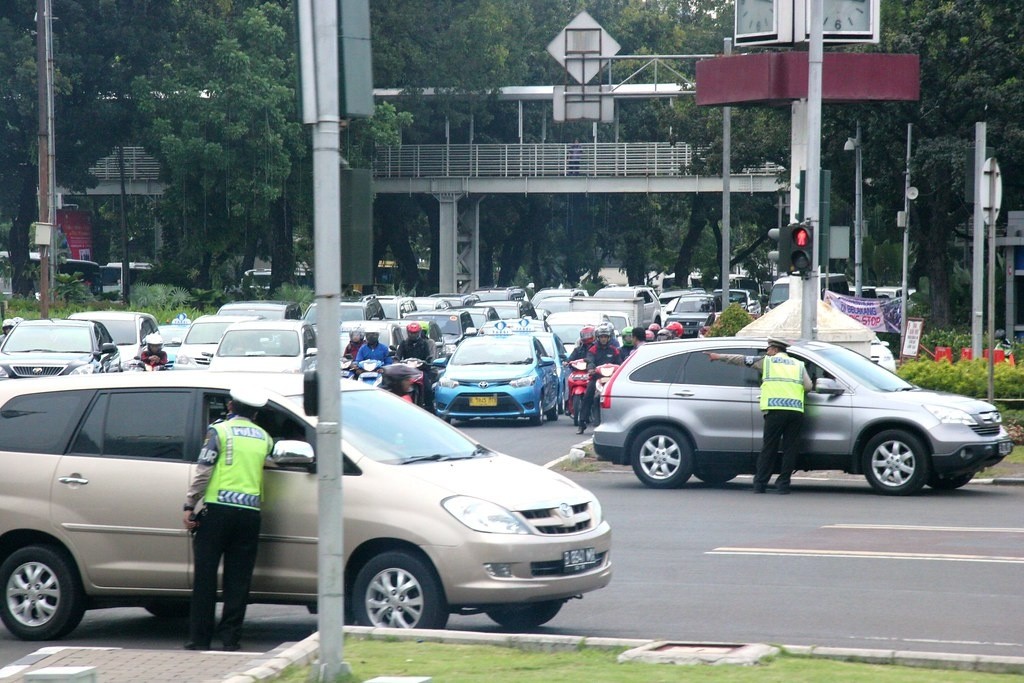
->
[0,317,25,347]
[342,325,367,362]
[702,335,813,495]
[395,319,437,417]
[561,322,686,434]
[141,332,168,366]
[354,325,393,374]
[183,379,276,652]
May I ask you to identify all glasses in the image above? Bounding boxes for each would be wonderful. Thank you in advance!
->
[3,327,13,330]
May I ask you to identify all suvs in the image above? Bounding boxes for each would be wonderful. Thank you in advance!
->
[593,336,1014,497]
[0,371,611,643]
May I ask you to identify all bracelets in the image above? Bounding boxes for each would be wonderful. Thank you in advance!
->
[184,502,195,511]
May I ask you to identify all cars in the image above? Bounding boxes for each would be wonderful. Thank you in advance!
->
[194,320,318,373]
[2,252,915,410]
[433,322,560,426]
[0,319,120,382]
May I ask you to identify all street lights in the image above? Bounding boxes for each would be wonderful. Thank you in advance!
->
[844,119,862,298]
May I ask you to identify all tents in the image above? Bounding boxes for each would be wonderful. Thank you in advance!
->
[738,291,872,359]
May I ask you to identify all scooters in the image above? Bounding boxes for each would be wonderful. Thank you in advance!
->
[562,359,620,426]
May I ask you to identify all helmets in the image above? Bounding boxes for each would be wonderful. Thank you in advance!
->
[13,316,23,323]
[348,327,364,340]
[2,319,16,330]
[406,322,421,339]
[580,317,683,347]
[147,334,164,351]
[364,326,380,341]
[418,322,429,337]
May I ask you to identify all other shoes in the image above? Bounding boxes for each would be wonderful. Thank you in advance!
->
[577,423,586,434]
[183,639,210,650]
[779,487,790,492]
[222,643,240,650]
[754,488,763,492]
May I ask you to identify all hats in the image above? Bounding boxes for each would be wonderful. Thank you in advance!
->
[766,337,791,351]
[230,383,269,411]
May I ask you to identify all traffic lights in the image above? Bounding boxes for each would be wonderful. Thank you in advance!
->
[790,223,814,271]
[768,226,790,272]
